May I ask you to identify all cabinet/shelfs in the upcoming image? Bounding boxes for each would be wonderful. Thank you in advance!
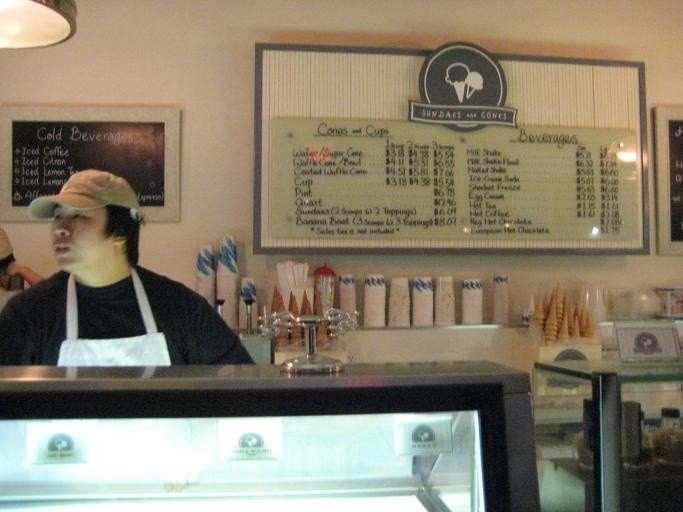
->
[535,357,680,511]
[1,363,535,510]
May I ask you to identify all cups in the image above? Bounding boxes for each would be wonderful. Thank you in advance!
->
[578,286,610,322]
[194,234,258,331]
[337,272,510,329]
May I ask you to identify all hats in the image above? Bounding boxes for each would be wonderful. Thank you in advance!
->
[27,168,142,222]
[0,227,13,261]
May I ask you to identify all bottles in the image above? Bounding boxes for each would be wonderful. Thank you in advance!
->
[312,261,336,313]
[660,407,681,429]
